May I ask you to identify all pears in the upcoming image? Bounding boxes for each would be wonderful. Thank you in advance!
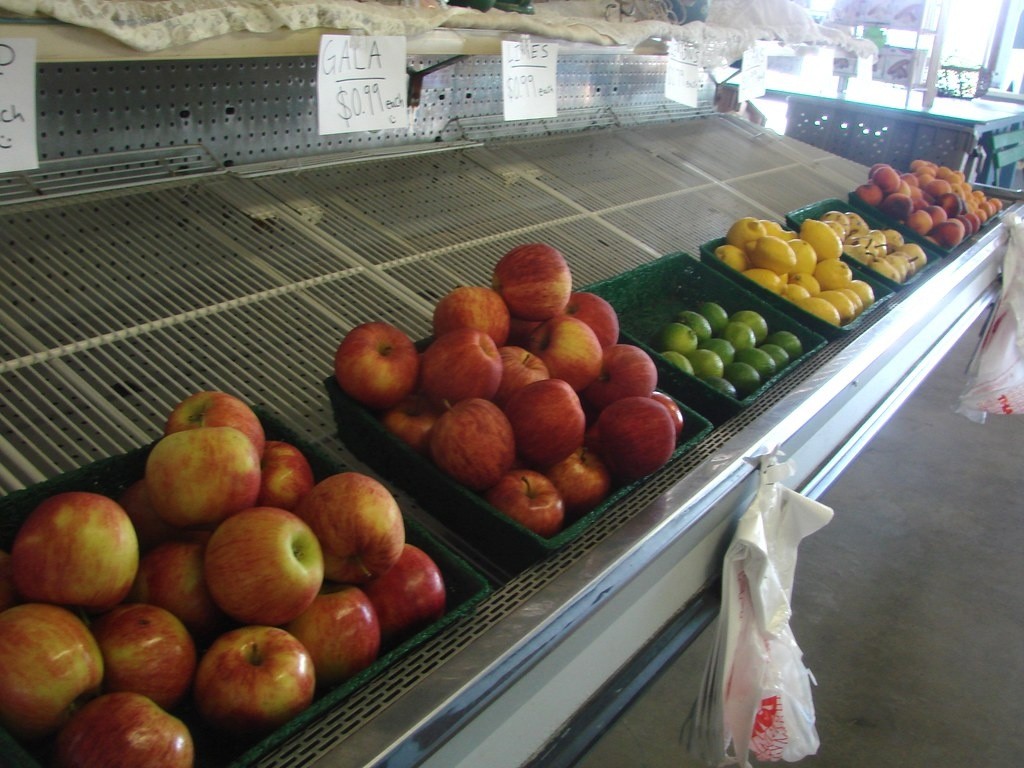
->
[822,208,928,283]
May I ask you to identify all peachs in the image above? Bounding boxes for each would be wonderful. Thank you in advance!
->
[854,160,983,254]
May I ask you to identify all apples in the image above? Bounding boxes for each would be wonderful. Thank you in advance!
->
[0,242,685,768]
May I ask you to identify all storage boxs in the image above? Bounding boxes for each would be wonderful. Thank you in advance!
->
[699,224,897,343]
[1,402,490,768]
[848,191,972,256]
[785,199,943,293]
[322,331,715,576]
[573,252,830,427]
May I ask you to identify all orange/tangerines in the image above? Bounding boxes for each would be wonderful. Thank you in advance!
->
[662,302,810,399]
[909,156,1004,223]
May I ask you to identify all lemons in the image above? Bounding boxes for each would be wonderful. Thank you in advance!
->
[707,212,873,325]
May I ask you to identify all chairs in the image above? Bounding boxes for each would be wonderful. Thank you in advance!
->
[988,129,1023,187]
